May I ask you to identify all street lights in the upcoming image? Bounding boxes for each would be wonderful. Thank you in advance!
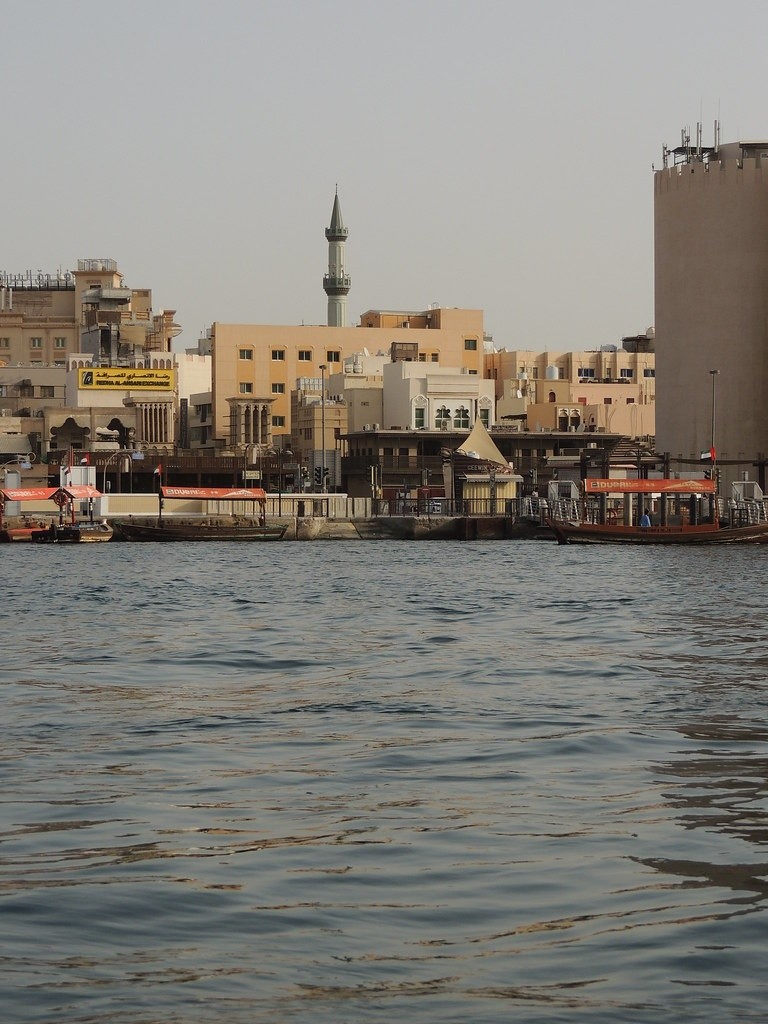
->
[709,369,718,480]
[320,364,329,492]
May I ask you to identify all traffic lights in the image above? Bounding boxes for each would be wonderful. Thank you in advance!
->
[323,468,328,477]
[704,469,712,481]
[714,469,722,483]
[315,467,321,486]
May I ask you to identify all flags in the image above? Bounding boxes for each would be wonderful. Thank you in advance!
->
[700,448,712,458]
[152,466,159,473]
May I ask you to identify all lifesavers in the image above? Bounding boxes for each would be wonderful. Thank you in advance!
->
[53,491,68,506]
[604,508,617,524]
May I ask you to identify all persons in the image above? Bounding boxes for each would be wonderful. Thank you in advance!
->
[532,487,539,497]
[639,508,651,526]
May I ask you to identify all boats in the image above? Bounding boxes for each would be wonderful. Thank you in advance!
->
[0,486,114,542]
[115,488,289,542]
[545,479,768,545]
